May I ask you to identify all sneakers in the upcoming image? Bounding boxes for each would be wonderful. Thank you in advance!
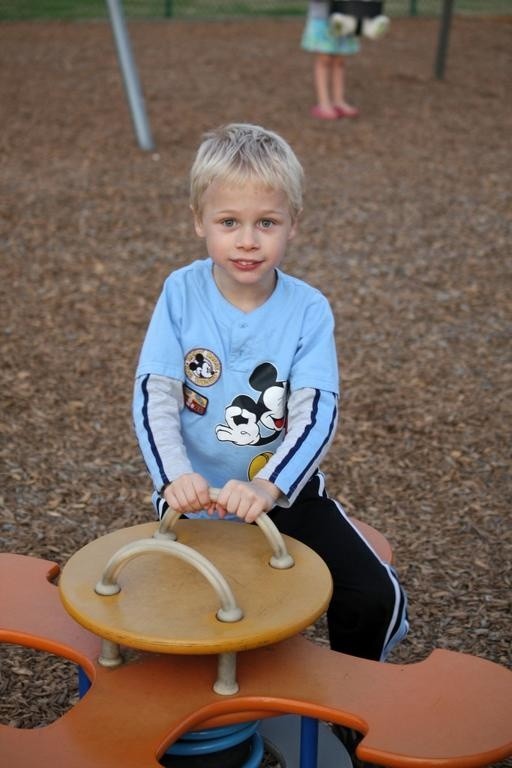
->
[313,103,359,120]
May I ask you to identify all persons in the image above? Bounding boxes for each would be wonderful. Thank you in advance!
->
[298,0,361,120]
[130,124,408,767]
